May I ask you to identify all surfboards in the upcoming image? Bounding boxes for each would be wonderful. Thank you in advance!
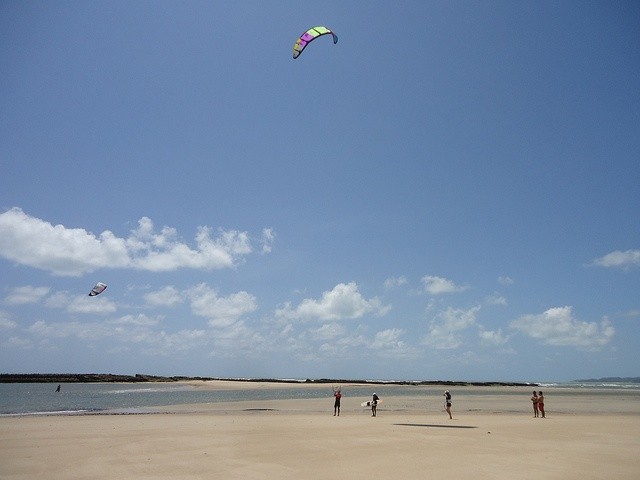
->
[361,399,384,407]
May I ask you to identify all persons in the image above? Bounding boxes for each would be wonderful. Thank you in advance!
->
[443,390,452,419]
[334,391,341,416]
[531,391,538,417]
[372,393,380,416]
[56,385,61,392]
[537,391,545,418]
